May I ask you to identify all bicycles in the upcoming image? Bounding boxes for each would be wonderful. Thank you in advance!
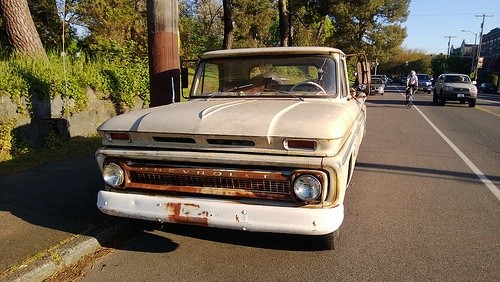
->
[406,86,416,109]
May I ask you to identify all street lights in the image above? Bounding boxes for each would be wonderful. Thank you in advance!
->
[462,29,478,81]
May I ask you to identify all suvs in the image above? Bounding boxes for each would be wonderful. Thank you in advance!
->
[433,73,478,107]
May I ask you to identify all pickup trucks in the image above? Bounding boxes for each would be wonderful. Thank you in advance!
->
[94,46,368,251]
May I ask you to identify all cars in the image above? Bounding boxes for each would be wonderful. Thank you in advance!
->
[416,73,432,91]
[371,73,393,95]
[478,82,498,94]
[400,77,407,85]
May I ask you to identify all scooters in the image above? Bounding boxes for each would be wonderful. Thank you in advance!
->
[422,78,434,94]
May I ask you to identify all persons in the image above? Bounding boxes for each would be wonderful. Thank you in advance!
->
[428,78,435,87]
[405,70,418,105]
